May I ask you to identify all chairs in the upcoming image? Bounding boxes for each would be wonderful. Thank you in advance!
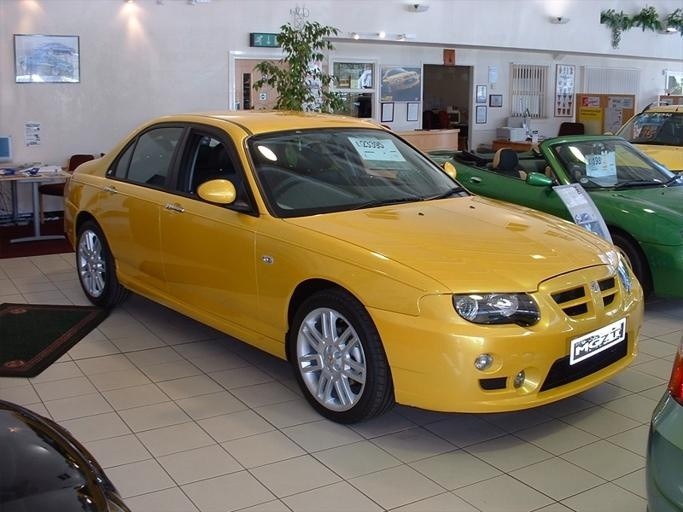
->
[558,122,584,137]
[492,147,521,178]
[35,154,95,224]
[210,140,361,214]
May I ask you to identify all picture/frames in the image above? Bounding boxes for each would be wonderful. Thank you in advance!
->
[378,62,422,123]
[13,33,83,83]
[476,84,503,124]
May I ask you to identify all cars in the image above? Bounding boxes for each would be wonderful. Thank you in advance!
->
[62,110,645,425]
[644,334,683,510]
[0,399,134,511]
[383,68,420,94]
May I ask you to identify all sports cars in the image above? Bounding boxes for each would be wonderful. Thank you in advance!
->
[426,134,683,306]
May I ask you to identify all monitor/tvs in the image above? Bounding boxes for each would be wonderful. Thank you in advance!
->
[448,112,460,124]
[0,136,12,161]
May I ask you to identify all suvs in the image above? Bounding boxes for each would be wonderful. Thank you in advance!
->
[612,101,682,182]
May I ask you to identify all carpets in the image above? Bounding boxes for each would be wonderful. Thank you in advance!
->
[0,299,111,377]
[0,216,74,260]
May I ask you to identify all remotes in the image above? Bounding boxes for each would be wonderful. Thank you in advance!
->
[30,168,38,174]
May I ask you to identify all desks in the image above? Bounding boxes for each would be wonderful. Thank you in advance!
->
[491,139,537,153]
[1,162,72,243]
